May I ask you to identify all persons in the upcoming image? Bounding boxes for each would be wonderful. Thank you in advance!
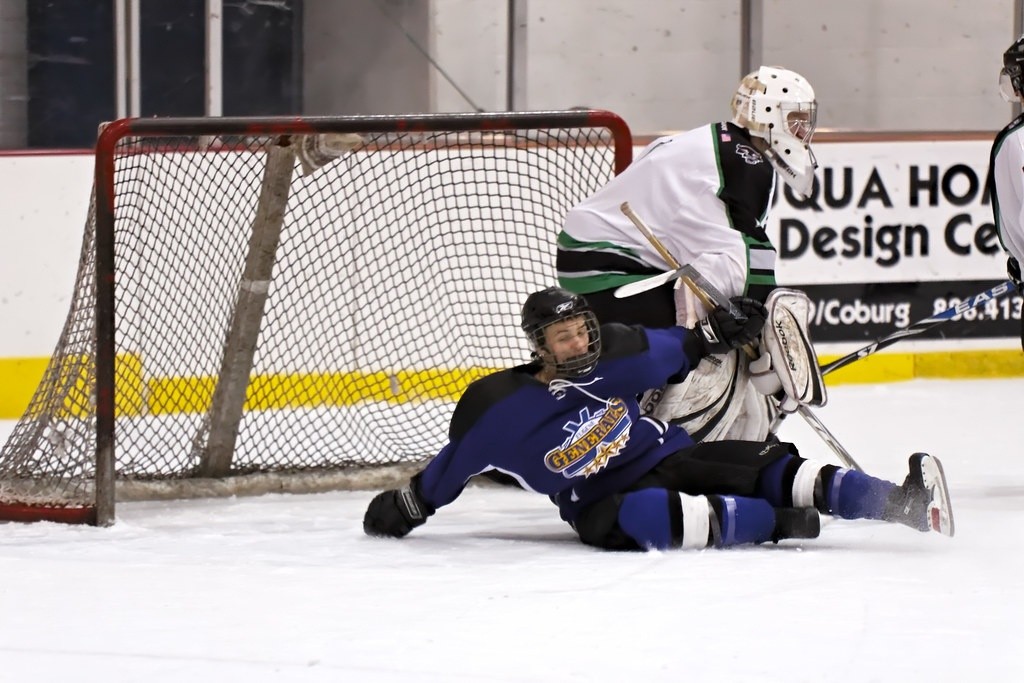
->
[556,66,818,443]
[988,36,1024,350]
[365,288,955,552]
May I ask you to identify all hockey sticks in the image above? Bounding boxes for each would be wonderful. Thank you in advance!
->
[819,281,1016,377]
[613,263,748,320]
[619,200,864,473]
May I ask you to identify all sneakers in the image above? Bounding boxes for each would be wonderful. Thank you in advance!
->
[883,453,956,536]
[771,506,824,544]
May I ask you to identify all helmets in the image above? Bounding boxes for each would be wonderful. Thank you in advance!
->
[727,66,819,200]
[521,286,601,379]
[998,34,1024,108]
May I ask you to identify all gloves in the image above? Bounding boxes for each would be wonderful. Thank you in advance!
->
[682,295,768,369]
[362,474,435,537]
[1005,258,1020,290]
